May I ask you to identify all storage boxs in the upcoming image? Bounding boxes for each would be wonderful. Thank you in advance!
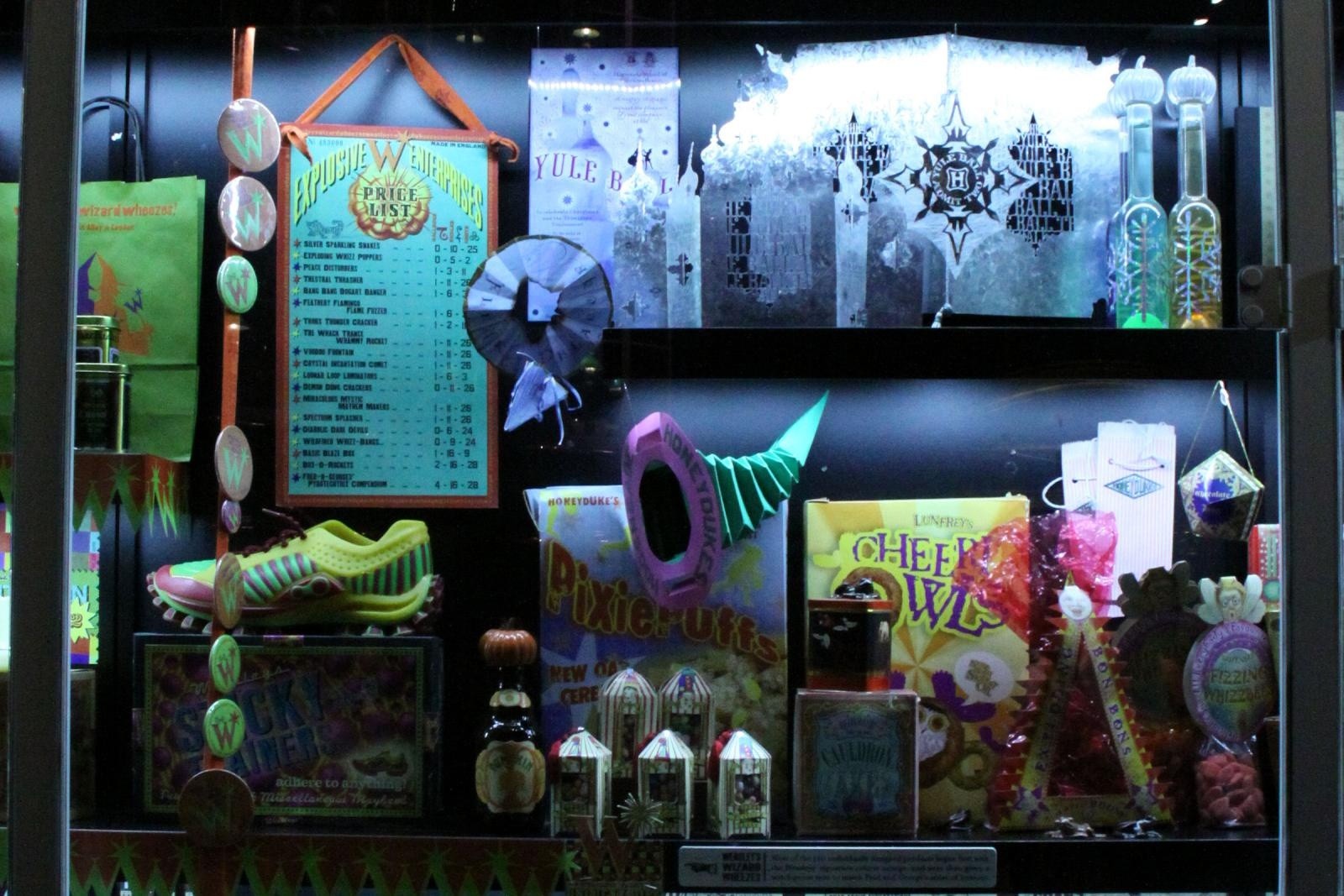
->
[129,622,441,826]
[805,596,899,695]
[788,679,923,845]
[517,477,790,805]
[790,485,1046,828]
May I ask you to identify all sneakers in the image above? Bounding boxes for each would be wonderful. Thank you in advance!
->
[138,507,444,632]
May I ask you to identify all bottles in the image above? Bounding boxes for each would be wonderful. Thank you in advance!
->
[473,664,547,838]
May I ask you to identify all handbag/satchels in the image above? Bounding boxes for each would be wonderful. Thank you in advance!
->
[0,93,206,464]
